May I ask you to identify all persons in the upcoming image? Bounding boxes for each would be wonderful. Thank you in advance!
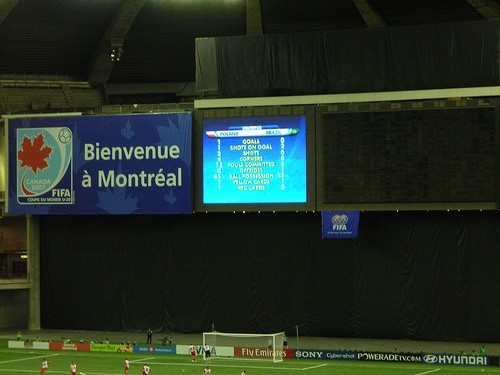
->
[122,328,247,375]
[267,337,272,348]
[461,345,488,356]
[283,334,288,349]
[16,331,110,375]
[395,349,398,353]
